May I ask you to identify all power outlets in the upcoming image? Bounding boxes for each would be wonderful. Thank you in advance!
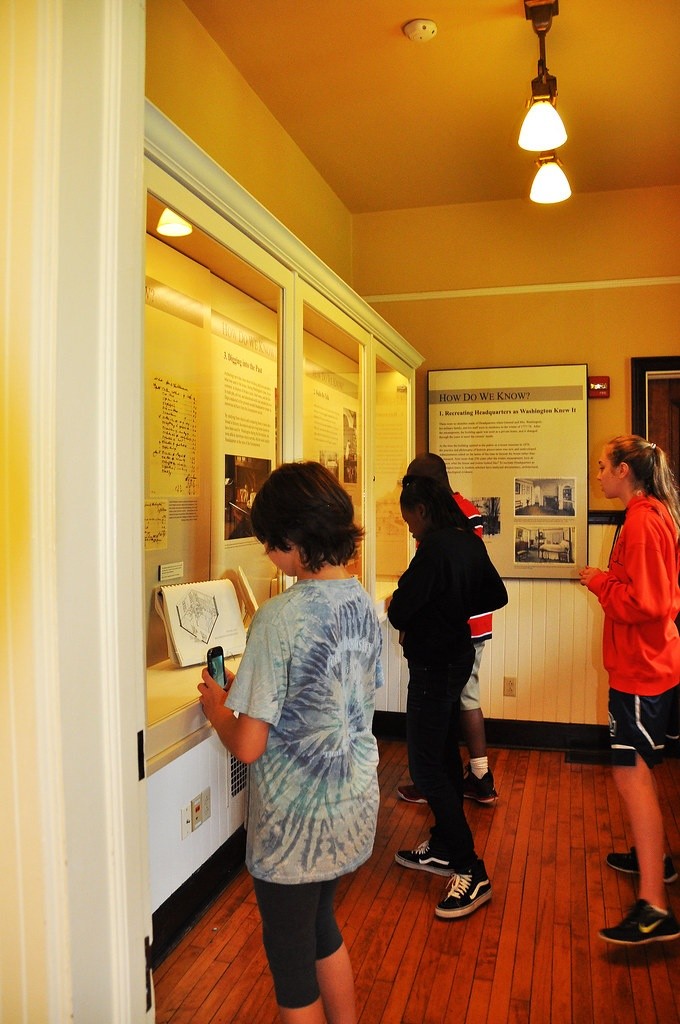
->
[503,677,516,696]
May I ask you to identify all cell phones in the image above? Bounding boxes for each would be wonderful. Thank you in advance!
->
[207,646,226,691]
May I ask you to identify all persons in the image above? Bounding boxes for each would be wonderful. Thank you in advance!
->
[386,477,507,918]
[395,453,498,803]
[579,436,680,944]
[197,462,385,1024]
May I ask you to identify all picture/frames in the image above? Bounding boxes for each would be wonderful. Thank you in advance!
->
[235,566,259,624]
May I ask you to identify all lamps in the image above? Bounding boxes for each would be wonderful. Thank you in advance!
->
[517,0,567,152]
[528,148,572,203]
[156,206,193,236]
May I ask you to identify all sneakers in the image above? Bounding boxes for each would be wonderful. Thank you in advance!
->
[606,846,679,883]
[395,840,454,877]
[461,762,497,802]
[599,899,680,946]
[397,783,428,804]
[434,860,491,919]
